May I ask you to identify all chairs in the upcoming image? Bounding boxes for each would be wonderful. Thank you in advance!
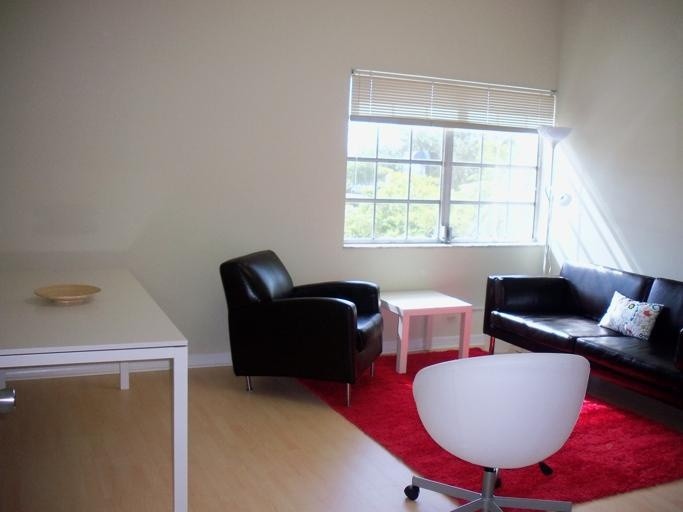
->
[219,250,383,406]
[403,353,592,512]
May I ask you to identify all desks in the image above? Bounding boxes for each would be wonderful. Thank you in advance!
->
[0,258,188,512]
[377,289,474,374]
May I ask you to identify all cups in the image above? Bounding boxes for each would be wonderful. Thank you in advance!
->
[439,225,453,244]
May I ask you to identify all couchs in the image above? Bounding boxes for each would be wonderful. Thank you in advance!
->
[483,261,683,411]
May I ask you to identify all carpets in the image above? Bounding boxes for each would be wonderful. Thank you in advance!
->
[296,346,683,512]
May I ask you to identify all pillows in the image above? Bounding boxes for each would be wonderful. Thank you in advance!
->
[597,291,664,341]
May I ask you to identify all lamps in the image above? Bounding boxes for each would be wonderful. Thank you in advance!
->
[536,124,572,276]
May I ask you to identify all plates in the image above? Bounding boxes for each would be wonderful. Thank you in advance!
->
[34,283,102,307]
